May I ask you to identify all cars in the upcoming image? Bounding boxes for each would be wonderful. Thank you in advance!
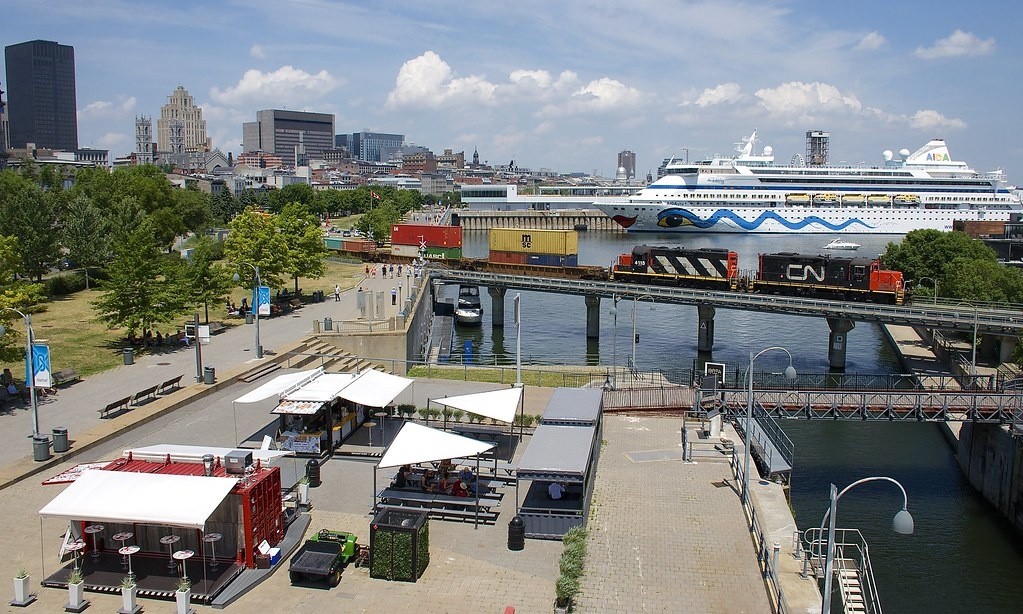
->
[319,222,340,237]
[354,229,362,237]
[343,231,351,237]
[58,258,82,271]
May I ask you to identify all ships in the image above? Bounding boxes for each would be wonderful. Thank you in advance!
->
[591,127,1023,236]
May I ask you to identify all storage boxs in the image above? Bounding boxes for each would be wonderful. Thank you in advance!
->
[267,548,281,565]
[323,225,578,266]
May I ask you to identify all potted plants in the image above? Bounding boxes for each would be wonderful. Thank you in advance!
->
[385,404,542,435]
[13,567,193,614]
[299,476,312,503]
[553,526,590,614]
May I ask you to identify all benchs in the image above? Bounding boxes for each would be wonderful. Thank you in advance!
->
[472,472,516,479]
[400,487,440,492]
[431,507,496,517]
[368,503,431,512]
[483,493,501,497]
[0,367,82,414]
[271,299,306,318]
[97,374,185,420]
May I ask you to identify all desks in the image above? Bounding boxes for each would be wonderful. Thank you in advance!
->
[370,455,518,526]
[64,525,222,588]
[363,403,397,447]
[451,426,503,458]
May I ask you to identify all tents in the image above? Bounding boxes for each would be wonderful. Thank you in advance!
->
[426,384,525,464]
[373,420,497,529]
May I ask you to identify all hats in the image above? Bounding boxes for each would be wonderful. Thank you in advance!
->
[460,483,467,489]
[464,467,469,472]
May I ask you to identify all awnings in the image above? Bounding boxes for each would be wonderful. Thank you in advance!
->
[122,443,297,483]
[333,368,415,446]
[39,469,241,595]
[231,365,322,448]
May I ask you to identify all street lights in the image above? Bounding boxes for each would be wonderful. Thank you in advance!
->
[412,259,417,279]
[406,270,411,299]
[0,307,39,438]
[398,281,403,314]
[953,301,978,381]
[740,346,798,502]
[231,261,260,358]
[609,292,619,389]
[818,477,914,614]
[631,295,657,373]
[916,276,937,306]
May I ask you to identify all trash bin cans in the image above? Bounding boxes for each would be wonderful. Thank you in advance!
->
[205,367,215,384]
[324,318,333,331]
[508,517,525,551]
[52,427,69,453]
[313,292,319,303]
[32,435,50,462]
[123,348,134,365]
[318,290,324,302]
[306,459,320,488]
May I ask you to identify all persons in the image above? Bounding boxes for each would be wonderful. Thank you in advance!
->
[225,296,283,318]
[393,464,477,497]
[365,265,369,278]
[371,266,376,278]
[358,287,368,292]
[382,264,413,279]
[391,287,397,305]
[277,288,303,297]
[128,330,191,347]
[549,482,565,500]
[335,285,340,302]
[7,380,57,406]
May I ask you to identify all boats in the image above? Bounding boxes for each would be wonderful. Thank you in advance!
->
[786,193,810,205]
[867,195,891,207]
[454,284,484,328]
[822,237,862,250]
[841,194,866,206]
[812,192,840,205]
[893,194,921,207]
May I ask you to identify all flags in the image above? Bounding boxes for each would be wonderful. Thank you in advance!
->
[371,191,381,199]
